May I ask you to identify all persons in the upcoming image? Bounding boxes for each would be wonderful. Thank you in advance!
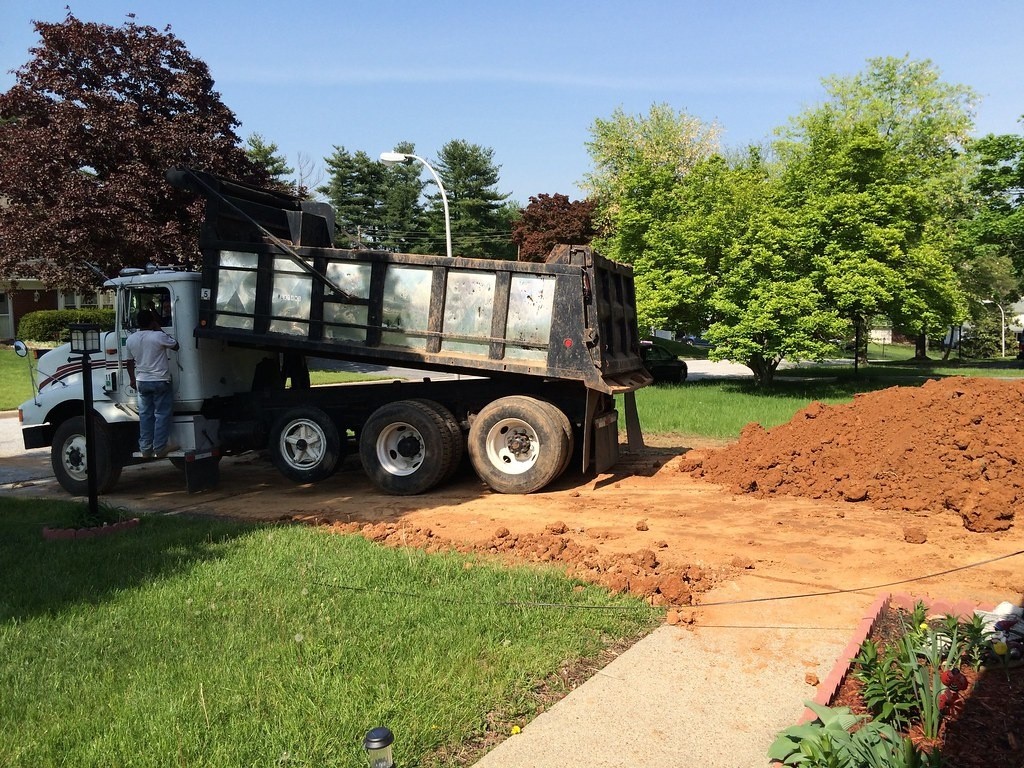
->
[149,301,172,327]
[126,309,180,457]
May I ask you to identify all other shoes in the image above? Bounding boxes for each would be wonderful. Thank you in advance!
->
[143,444,181,459]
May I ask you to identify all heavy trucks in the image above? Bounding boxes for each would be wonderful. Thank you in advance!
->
[12,163,653,495]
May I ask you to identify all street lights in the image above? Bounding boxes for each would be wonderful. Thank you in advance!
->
[381,151,452,258]
[982,300,1005,357]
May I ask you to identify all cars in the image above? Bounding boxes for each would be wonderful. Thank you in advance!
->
[684,331,717,349]
[640,341,689,384]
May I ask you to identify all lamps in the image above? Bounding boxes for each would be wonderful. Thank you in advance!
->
[32,289,41,302]
[66,318,103,360]
